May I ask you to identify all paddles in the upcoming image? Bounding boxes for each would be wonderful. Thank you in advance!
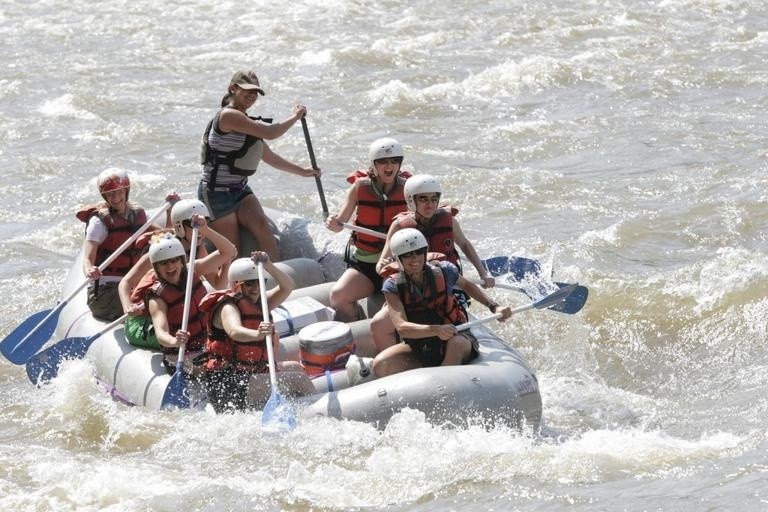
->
[258,255,297,434]
[160,217,199,410]
[0,197,176,365]
[336,221,540,281]
[464,276,588,315]
[26,303,145,388]
[454,282,580,331]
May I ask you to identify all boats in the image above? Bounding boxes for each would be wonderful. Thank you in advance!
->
[59,208,543,434]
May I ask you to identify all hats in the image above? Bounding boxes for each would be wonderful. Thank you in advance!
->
[230,69,265,96]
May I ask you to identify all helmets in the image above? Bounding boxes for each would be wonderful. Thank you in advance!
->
[149,199,267,289]
[97,167,130,203]
[369,137,441,271]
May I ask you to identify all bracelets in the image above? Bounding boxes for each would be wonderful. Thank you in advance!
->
[490,303,498,312]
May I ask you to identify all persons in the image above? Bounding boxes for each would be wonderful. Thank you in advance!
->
[117,199,239,350]
[199,69,321,289]
[202,251,318,414]
[76,168,183,321]
[373,228,511,379]
[370,174,496,353]
[130,214,236,377]
[325,137,458,322]
